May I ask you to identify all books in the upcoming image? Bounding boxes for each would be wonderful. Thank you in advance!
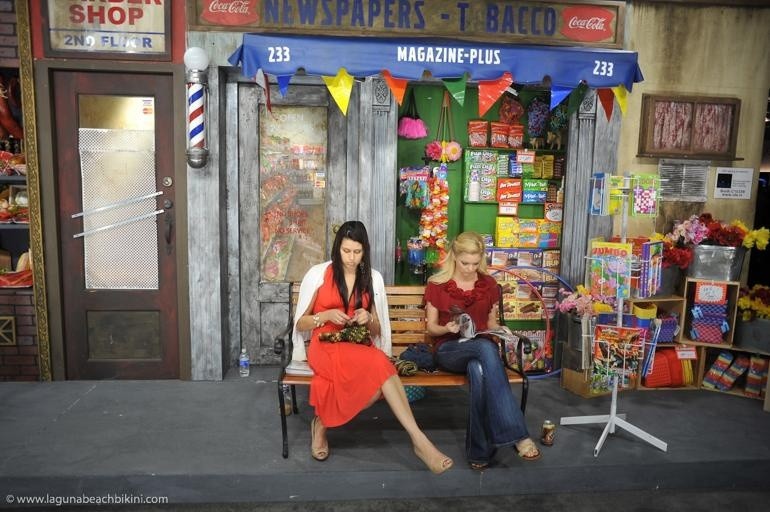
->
[591,172,661,217]
[589,238,665,298]
[453,313,512,343]
[581,311,661,392]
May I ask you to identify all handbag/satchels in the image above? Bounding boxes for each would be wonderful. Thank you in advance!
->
[401,342,434,372]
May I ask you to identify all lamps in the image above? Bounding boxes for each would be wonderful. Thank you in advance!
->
[180,46,211,168]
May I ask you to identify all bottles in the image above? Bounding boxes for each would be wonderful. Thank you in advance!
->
[541,415,556,446]
[239,346,251,377]
[278,383,291,415]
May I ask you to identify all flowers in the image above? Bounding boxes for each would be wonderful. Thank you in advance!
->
[735,284,770,322]
[653,211,770,270]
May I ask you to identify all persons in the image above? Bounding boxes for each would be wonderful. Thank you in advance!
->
[423,231,542,471]
[296,221,453,475]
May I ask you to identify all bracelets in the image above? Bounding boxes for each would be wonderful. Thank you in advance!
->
[312,312,325,328]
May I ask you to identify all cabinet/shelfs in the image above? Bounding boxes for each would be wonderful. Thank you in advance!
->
[0,66,33,290]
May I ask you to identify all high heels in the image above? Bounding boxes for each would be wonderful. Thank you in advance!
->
[310,416,330,461]
[412,446,454,476]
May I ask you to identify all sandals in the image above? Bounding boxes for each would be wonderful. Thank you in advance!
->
[470,460,489,470]
[512,436,542,463]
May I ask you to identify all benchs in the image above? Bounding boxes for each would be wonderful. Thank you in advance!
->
[272,281,533,458]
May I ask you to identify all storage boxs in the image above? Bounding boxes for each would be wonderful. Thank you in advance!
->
[686,244,747,281]
[680,277,740,349]
[638,343,702,390]
[699,344,770,401]
[623,294,684,346]
